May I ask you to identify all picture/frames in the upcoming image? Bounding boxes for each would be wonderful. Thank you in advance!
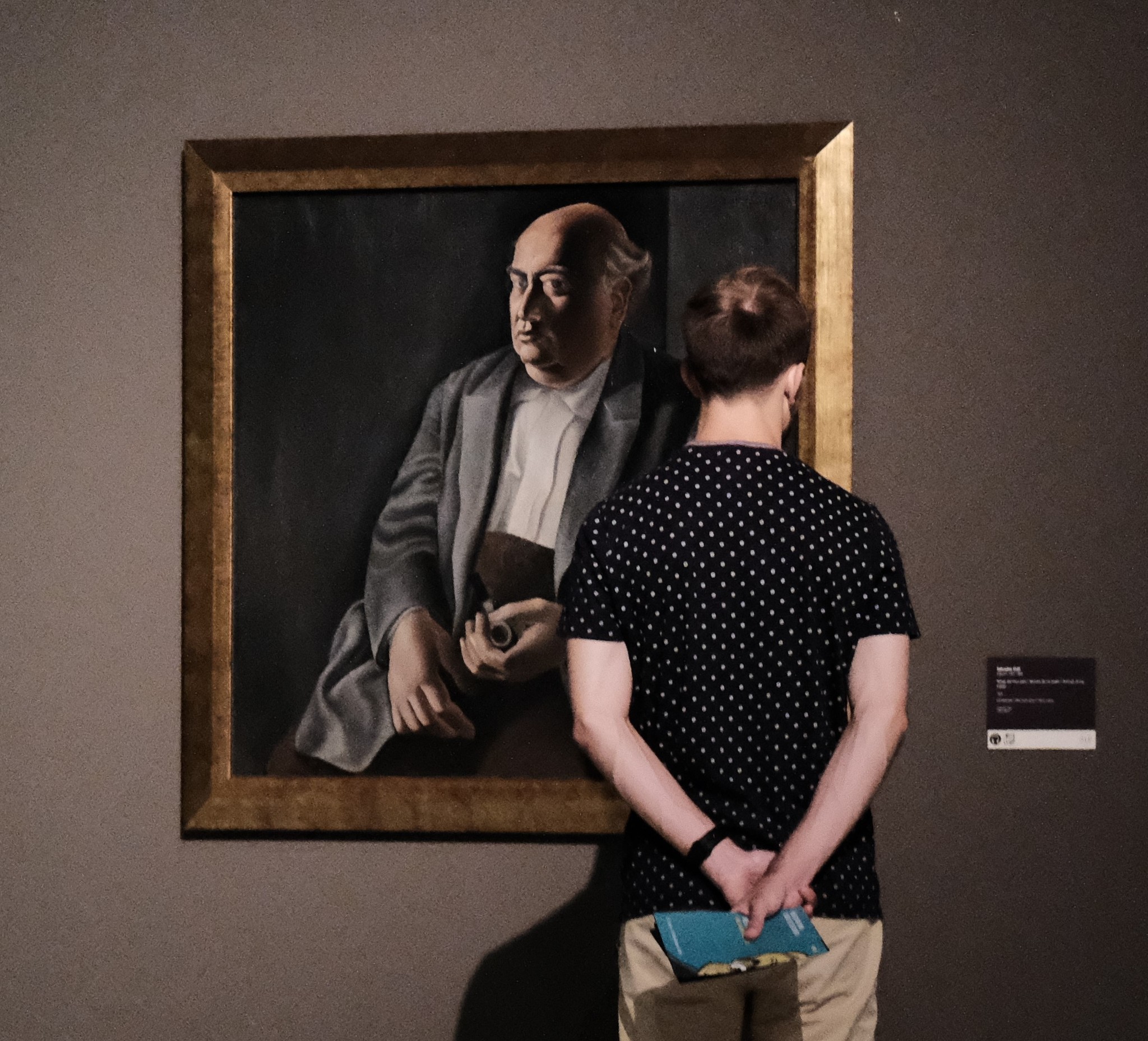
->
[180,120,856,836]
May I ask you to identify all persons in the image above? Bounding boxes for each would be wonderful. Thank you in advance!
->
[551,264,922,1041]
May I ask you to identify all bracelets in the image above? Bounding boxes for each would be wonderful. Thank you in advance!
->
[683,826,732,874]
